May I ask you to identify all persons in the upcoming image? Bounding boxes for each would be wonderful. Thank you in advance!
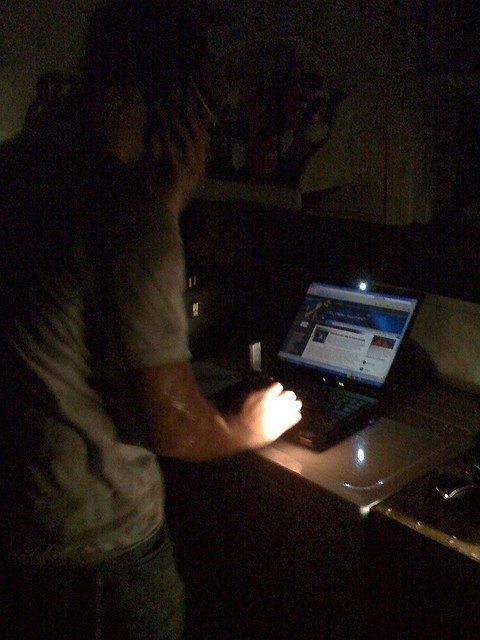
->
[0,89,302,636]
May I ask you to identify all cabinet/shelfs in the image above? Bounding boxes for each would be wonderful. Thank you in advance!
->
[190,0,440,228]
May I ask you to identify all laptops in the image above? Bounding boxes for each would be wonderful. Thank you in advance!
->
[213,279,420,452]
